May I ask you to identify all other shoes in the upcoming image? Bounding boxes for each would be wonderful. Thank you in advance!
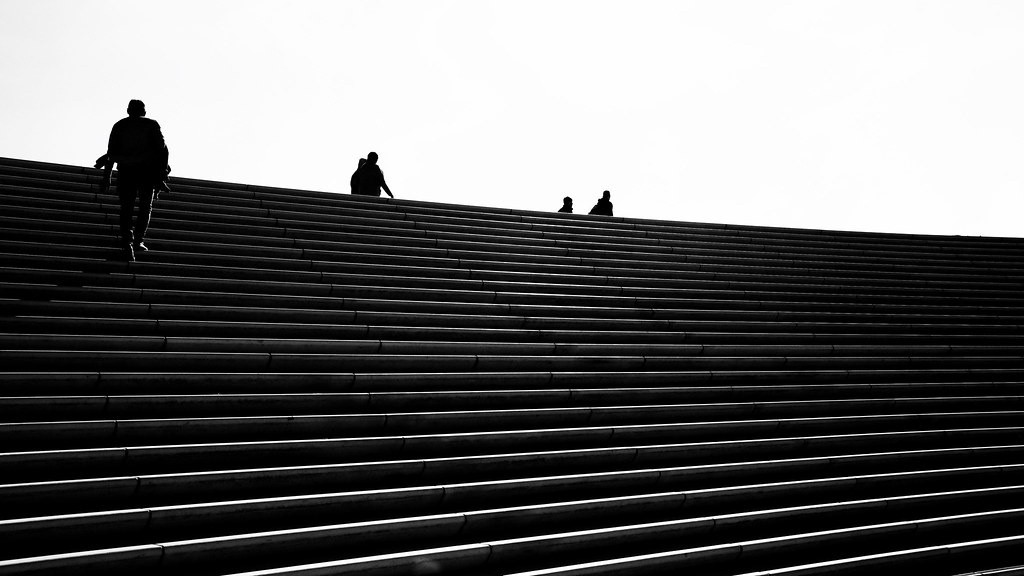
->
[133,240,149,252]
[107,244,136,262]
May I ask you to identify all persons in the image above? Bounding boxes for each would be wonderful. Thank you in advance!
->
[558,197,573,213]
[94,99,171,262]
[588,191,614,216]
[350,152,394,199]
